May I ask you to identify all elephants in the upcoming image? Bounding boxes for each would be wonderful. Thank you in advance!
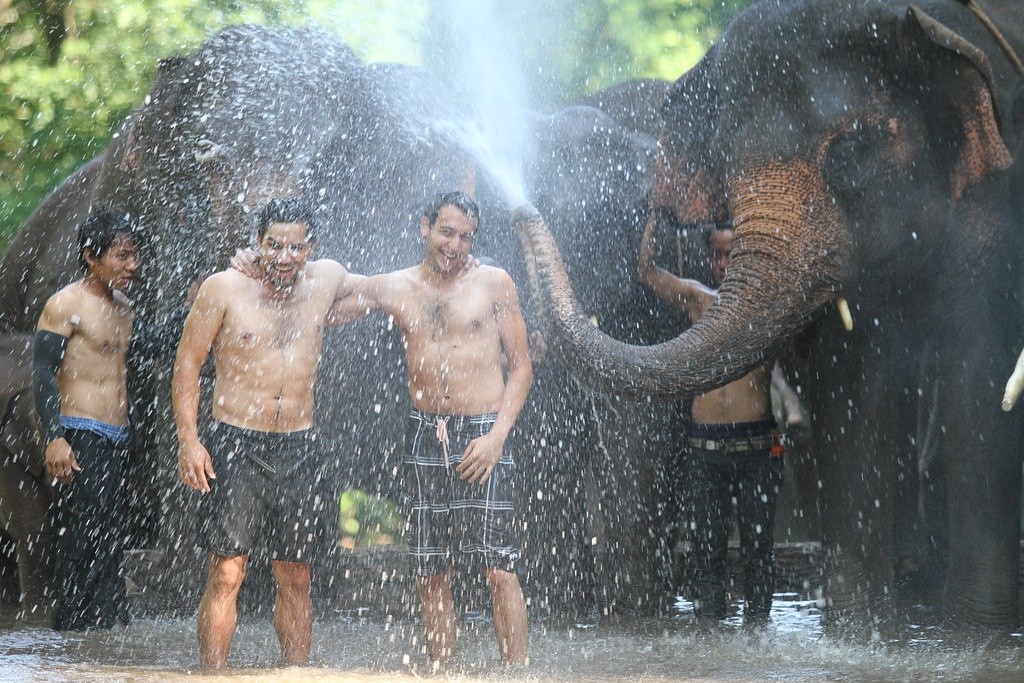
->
[1,2,1023,683]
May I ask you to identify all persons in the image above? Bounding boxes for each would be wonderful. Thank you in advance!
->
[172,187,534,674]
[636,197,811,624]
[32,208,144,632]
